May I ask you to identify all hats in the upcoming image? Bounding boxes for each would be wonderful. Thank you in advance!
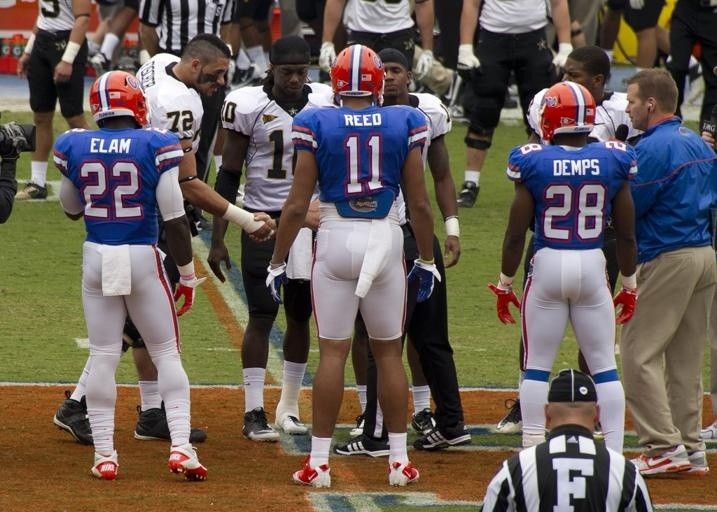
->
[546,368,599,402]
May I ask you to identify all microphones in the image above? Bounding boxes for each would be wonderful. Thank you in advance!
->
[615,124,629,142]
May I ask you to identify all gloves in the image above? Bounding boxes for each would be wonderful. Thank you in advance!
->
[547,43,574,80]
[317,41,336,75]
[2,121,25,161]
[414,49,434,82]
[172,276,208,317]
[612,288,636,325]
[455,44,484,85]
[486,282,520,326]
[406,258,442,303]
[264,259,289,304]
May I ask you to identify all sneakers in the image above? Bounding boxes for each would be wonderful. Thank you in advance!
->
[90,449,119,482]
[132,400,206,444]
[442,70,463,110]
[239,406,308,443]
[456,181,479,209]
[333,409,471,457]
[167,443,208,481]
[90,54,110,79]
[698,422,716,443]
[15,182,48,200]
[495,399,522,435]
[291,464,330,489]
[674,450,711,477]
[52,389,94,445]
[626,445,693,477]
[388,462,420,486]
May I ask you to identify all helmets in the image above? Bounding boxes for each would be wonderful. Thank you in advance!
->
[91,69,148,129]
[540,81,596,140]
[329,44,385,107]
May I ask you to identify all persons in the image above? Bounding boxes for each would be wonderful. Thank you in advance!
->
[1,1,716,512]
